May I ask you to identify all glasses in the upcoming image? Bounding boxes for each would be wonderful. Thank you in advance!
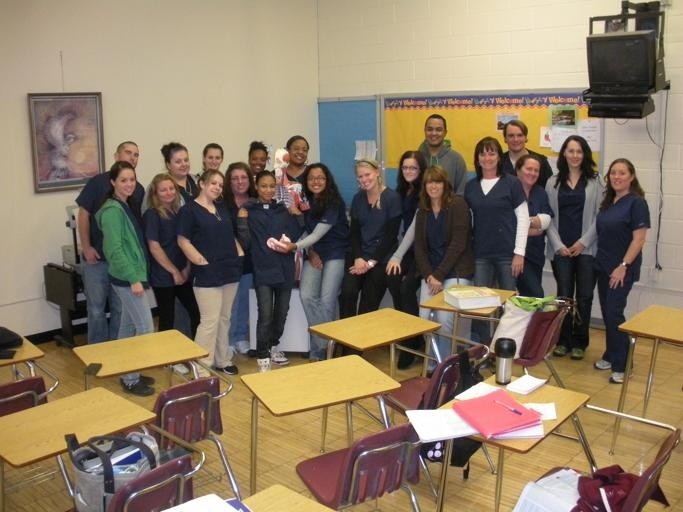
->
[402,166,419,173]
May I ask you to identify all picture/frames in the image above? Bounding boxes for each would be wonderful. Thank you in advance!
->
[27,92,104,193]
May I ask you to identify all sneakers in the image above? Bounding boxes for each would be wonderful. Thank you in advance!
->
[609,371,633,383]
[120,372,157,396]
[594,359,613,369]
[398,341,423,370]
[163,362,189,375]
[271,342,291,365]
[215,366,240,376]
[554,345,570,356]
[571,348,585,359]
[249,350,271,372]
[229,339,251,361]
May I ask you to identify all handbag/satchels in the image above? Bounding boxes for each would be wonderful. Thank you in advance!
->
[66,428,163,512]
[491,295,570,361]
[418,348,484,465]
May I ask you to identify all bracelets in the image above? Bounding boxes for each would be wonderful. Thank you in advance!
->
[619,260,631,268]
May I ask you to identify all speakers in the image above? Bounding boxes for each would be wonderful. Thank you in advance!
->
[634,2,660,31]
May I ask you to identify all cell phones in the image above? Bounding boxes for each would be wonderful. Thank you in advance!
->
[83,362,102,377]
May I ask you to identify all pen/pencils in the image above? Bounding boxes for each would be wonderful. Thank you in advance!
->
[495,400,522,415]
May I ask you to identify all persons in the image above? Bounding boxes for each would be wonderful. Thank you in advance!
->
[142,134,351,373]
[593,158,651,384]
[336,113,551,368]
[74,140,145,345]
[94,161,156,397]
[543,134,609,360]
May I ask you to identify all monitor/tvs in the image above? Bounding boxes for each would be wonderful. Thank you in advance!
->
[587,32,665,94]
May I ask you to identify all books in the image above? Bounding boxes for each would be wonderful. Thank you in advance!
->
[83,447,142,474]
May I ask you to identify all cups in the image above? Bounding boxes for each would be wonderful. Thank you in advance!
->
[494,337,516,386]
